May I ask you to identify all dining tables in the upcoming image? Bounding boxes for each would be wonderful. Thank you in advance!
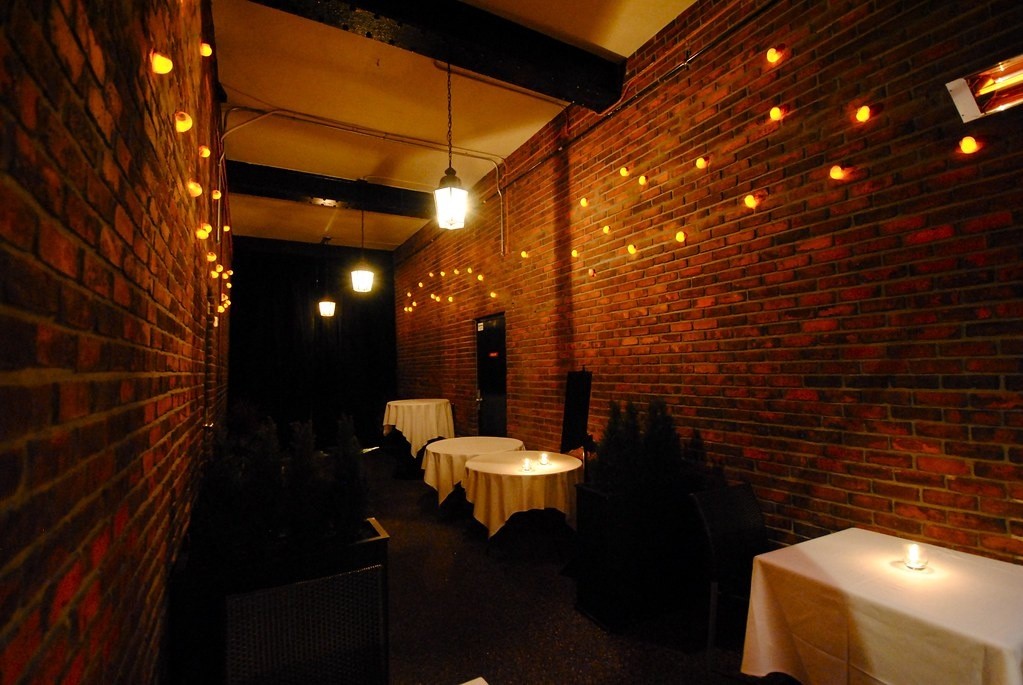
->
[461,448,582,540]
[419,435,526,507]
[383,398,455,479]
[741,525,1023,685]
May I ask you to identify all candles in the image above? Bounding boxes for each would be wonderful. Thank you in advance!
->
[540,453,548,465]
[904,542,922,568]
[523,457,531,471]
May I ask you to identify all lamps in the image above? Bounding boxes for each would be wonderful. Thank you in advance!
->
[351,181,375,293]
[433,66,468,233]
[318,236,337,318]
